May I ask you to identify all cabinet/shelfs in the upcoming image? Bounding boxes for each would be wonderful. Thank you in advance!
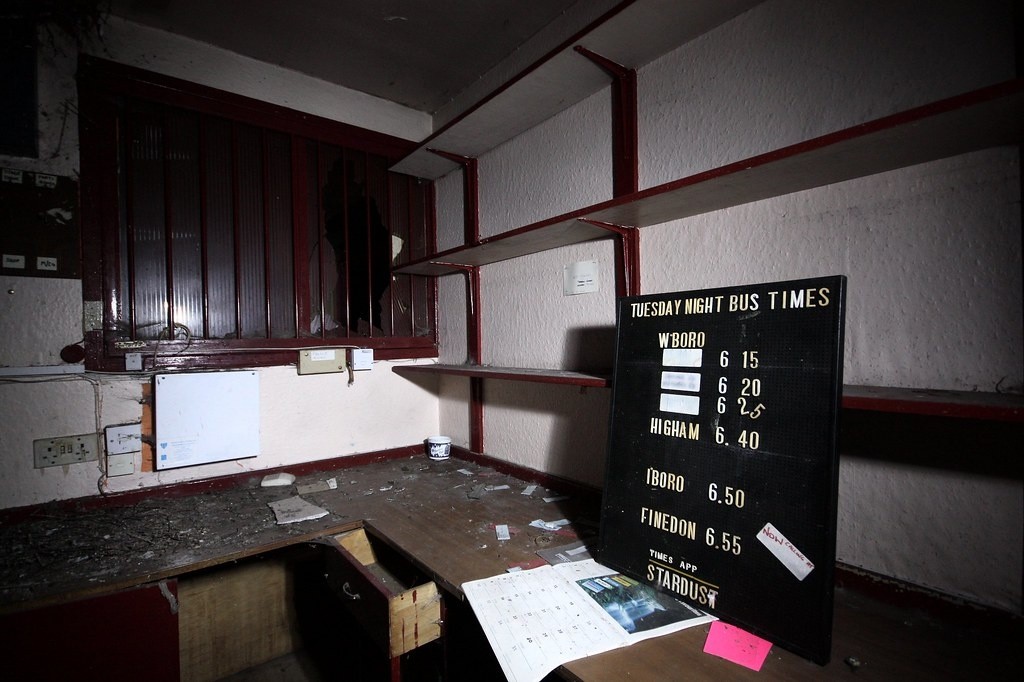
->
[388,0,1024,422]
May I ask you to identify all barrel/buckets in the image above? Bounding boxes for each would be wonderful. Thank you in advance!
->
[428,435,451,460]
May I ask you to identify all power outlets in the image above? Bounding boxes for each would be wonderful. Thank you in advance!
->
[33,435,98,470]
[107,424,141,455]
